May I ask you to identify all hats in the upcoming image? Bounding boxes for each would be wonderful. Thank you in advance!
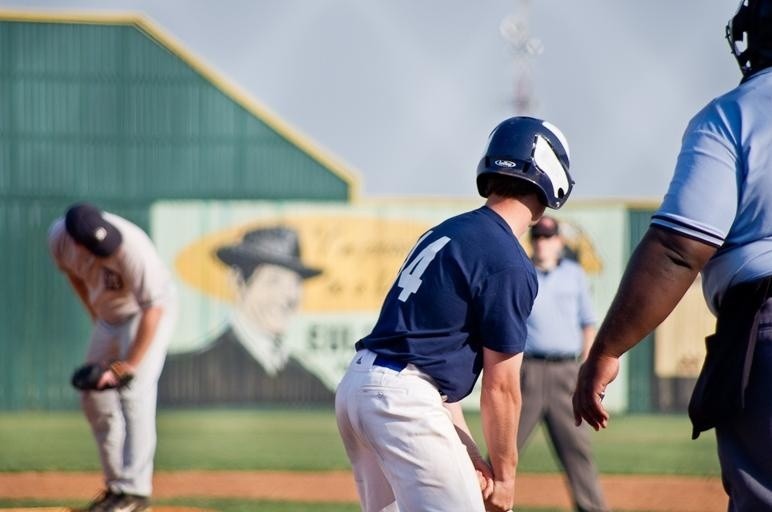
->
[532,217,558,236]
[215,227,323,280]
[65,203,122,257]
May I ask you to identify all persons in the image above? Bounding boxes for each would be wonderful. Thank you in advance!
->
[485,216,608,512]
[333,116,574,512]
[46,202,171,512]
[571,0,772,512]
[155,226,341,409]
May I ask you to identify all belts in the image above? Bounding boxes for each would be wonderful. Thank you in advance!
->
[356,353,408,372]
[533,351,576,363]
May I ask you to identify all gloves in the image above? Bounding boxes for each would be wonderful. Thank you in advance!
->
[97,361,136,391]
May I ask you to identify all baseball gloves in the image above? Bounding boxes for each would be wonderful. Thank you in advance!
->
[69,363,132,391]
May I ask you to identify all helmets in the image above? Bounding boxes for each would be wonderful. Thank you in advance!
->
[476,115,575,210]
[724,0,771,73]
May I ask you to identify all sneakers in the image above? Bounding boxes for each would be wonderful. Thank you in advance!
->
[88,490,149,512]
